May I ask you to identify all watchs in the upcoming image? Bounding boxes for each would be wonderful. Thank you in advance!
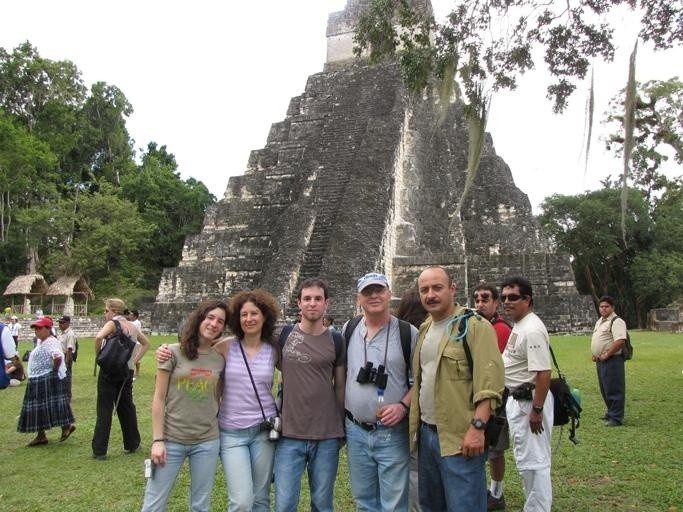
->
[532,406,543,413]
[471,420,487,430]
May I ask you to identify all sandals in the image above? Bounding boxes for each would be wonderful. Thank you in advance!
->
[60,424,76,442]
[28,434,47,446]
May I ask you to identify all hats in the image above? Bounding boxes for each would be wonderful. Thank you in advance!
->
[56,316,70,322]
[30,317,52,328]
[357,272,389,292]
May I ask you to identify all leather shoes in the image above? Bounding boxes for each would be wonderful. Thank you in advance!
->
[487,489,506,510]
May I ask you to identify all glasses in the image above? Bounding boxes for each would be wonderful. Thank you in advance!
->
[500,292,525,302]
[473,294,495,299]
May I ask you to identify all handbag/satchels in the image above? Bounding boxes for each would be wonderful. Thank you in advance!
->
[548,378,582,426]
[96,320,135,370]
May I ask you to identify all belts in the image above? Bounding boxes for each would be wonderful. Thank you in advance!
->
[342,408,378,432]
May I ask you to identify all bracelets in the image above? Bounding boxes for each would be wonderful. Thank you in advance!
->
[606,352,610,358]
[398,400,412,410]
[154,438,165,442]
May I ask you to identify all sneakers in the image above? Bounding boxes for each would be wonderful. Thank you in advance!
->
[599,413,620,426]
[124,444,141,453]
[92,452,106,459]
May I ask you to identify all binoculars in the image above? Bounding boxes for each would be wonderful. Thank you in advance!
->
[357,362,388,390]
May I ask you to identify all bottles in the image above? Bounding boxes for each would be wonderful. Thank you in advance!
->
[376,389,392,444]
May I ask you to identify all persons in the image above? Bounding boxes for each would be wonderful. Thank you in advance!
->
[341,273,419,512]
[410,266,504,512]
[155,289,283,512]
[590,295,627,426]
[474,286,513,512]
[0,299,149,459]
[323,316,337,331]
[142,298,227,512]
[273,279,346,512]
[501,278,556,512]
[396,284,431,512]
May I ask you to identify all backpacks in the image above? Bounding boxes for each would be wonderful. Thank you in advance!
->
[610,316,633,361]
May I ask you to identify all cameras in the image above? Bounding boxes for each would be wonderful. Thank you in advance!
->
[145,459,152,478]
[268,417,282,442]
